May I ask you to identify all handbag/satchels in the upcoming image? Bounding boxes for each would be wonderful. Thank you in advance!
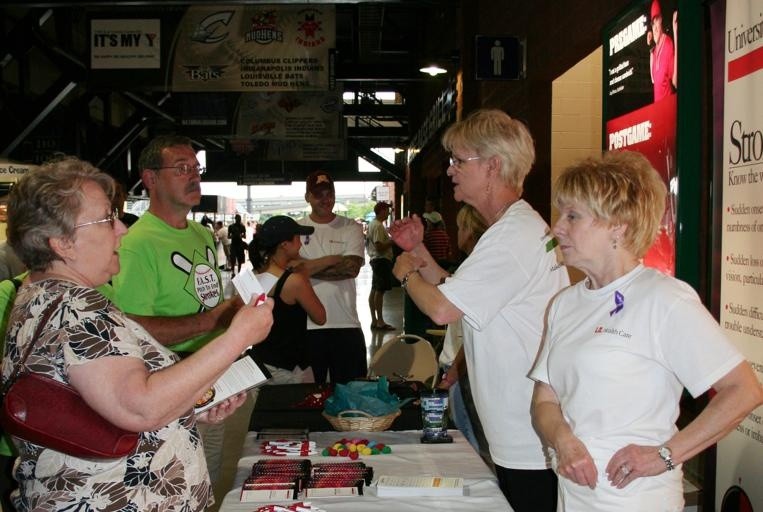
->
[3,297,138,463]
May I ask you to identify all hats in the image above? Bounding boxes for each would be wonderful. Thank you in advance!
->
[256,214,315,247]
[374,201,393,212]
[305,172,335,193]
[648,1,664,19]
[418,210,443,223]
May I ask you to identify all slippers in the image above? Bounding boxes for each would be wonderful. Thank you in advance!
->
[370,320,394,331]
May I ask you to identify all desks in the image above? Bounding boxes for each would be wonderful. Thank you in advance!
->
[219,383,515,512]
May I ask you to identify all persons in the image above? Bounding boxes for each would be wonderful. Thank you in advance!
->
[215,221,238,271]
[228,215,247,277]
[367,202,401,330]
[112,135,224,494]
[0,156,275,512]
[1,240,28,281]
[422,211,452,275]
[424,196,443,233]
[387,108,572,512]
[248,215,326,384]
[0,269,31,457]
[435,203,489,352]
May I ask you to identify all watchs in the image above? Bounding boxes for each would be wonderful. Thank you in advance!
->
[657,444,674,470]
[292,170,366,382]
[441,372,455,385]
[402,269,419,294]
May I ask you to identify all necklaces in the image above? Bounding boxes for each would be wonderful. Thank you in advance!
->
[487,198,521,225]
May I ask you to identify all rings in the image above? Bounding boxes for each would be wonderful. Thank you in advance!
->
[527,151,763,511]
[621,464,630,476]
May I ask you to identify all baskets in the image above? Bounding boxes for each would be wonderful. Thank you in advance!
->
[318,407,401,436]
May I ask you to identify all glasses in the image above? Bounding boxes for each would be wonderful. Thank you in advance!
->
[148,162,206,176]
[447,155,482,169]
[70,208,122,231]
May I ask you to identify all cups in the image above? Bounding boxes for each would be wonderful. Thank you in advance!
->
[419,388,449,443]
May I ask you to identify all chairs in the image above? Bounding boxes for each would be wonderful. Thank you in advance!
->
[368,335,439,387]
